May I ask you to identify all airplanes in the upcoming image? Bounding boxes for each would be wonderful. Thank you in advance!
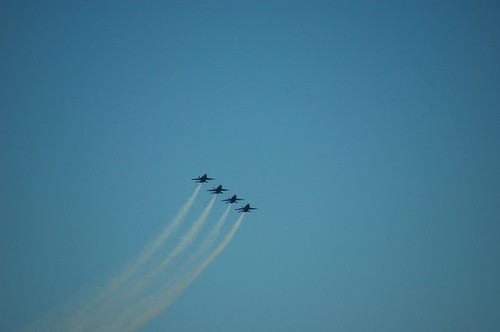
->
[191,173,257,215]
